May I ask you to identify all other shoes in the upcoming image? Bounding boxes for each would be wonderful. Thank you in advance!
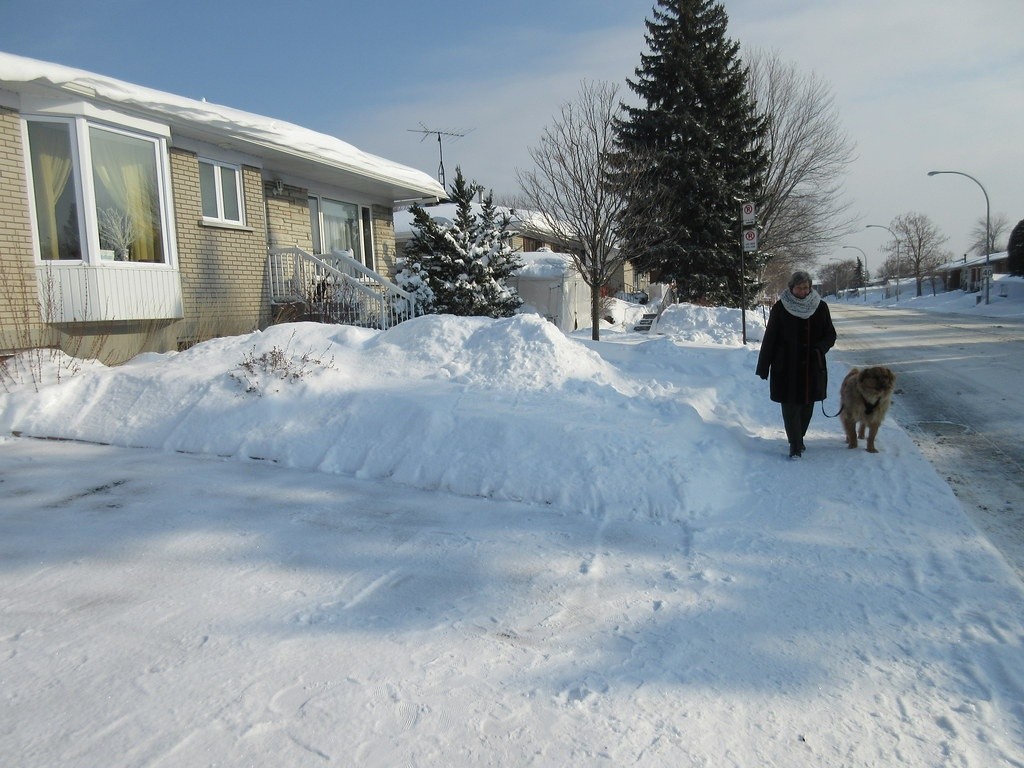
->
[790,443,801,460]
[801,444,806,452]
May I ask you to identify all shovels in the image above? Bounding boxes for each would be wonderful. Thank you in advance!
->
[314,248,348,301]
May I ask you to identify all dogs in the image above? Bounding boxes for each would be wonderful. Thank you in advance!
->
[840,366,897,453]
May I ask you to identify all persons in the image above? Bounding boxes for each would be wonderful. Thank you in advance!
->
[755,271,837,459]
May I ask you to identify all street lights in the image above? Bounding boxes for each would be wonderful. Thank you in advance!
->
[928,170,990,305]
[866,225,900,301]
[830,258,848,301]
[842,246,866,302]
